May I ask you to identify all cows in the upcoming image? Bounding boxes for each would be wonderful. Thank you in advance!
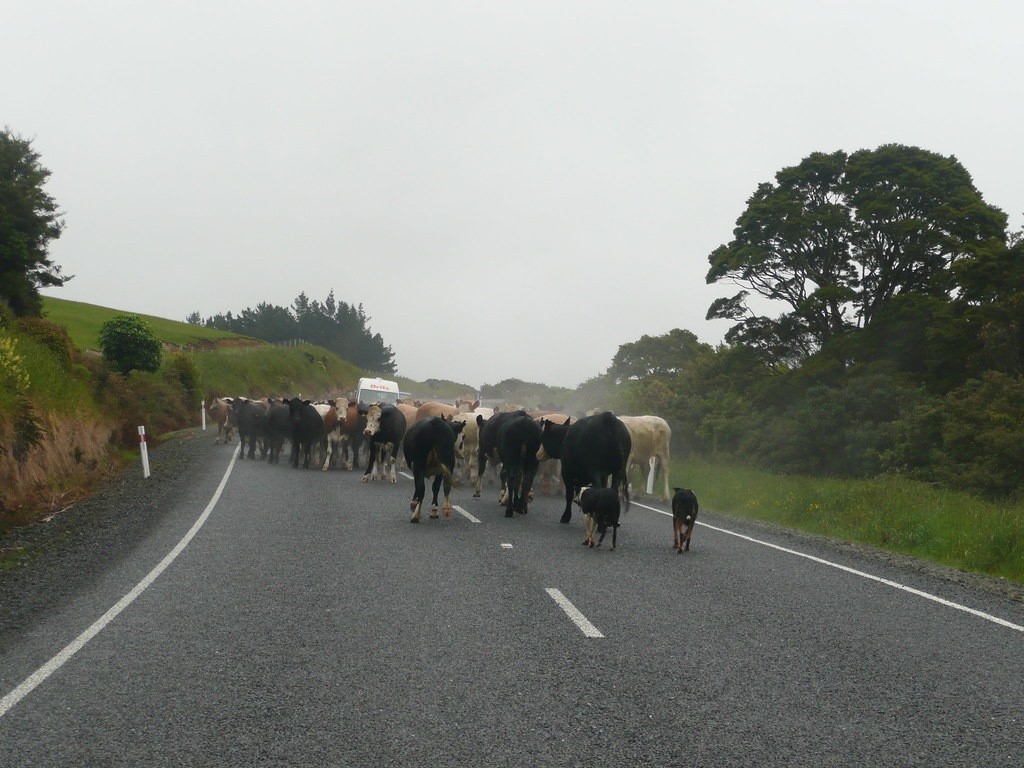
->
[206,396,672,533]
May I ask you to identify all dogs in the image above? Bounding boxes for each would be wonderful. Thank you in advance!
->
[574,482,621,551]
[671,487,699,554]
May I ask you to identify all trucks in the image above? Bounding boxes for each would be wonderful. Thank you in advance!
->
[354,377,399,408]
[398,391,412,401]
